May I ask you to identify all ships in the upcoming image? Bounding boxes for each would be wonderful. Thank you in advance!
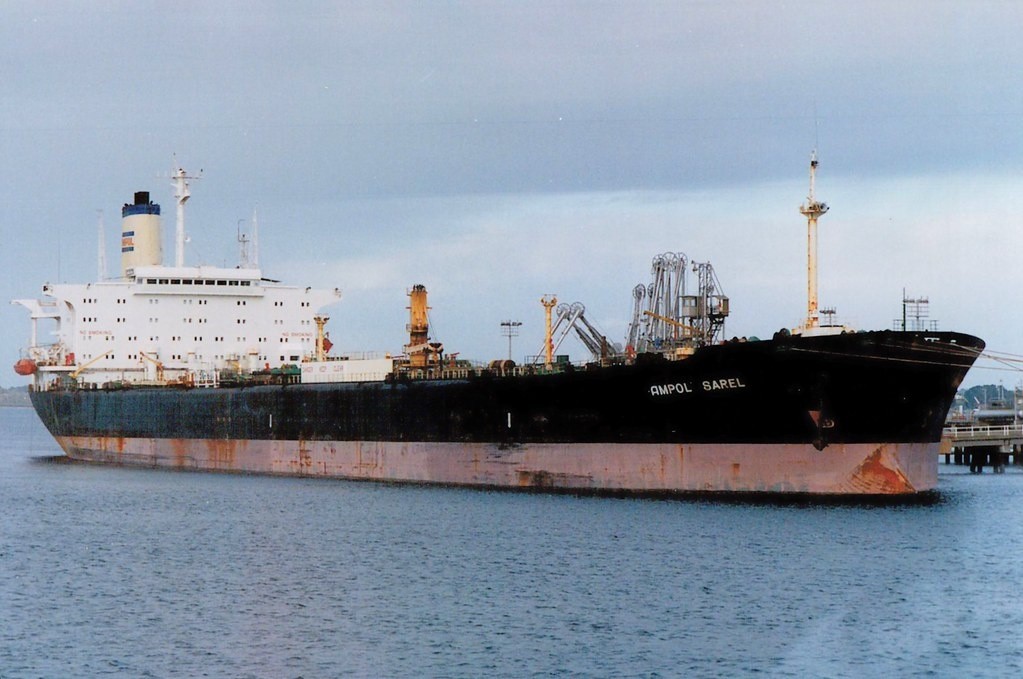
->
[15,167,989,505]
